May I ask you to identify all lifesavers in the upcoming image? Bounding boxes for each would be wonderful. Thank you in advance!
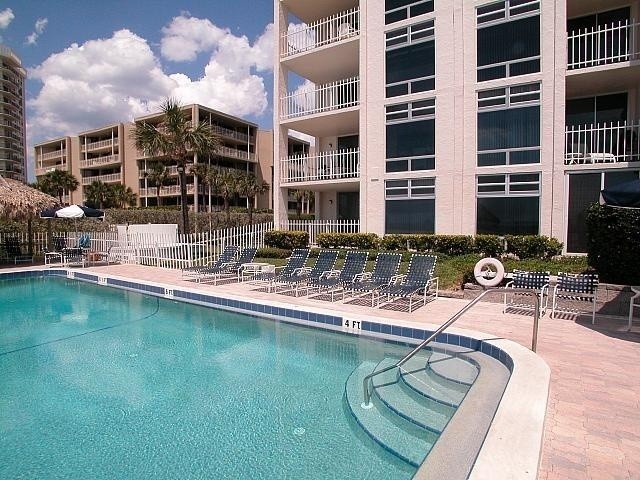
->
[474,257,506,286]
[78,235,90,247]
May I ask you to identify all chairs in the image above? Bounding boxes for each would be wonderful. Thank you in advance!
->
[43,230,116,267]
[4,237,33,265]
[628,286,640,330]
[552,271,599,325]
[338,23,357,41]
[504,269,550,319]
[179,245,439,313]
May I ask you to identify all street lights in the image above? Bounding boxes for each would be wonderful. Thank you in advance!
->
[177,163,183,233]
[297,201,302,216]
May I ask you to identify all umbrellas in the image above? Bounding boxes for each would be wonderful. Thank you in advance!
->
[36,203,105,248]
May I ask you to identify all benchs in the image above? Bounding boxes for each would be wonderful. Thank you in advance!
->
[587,153,616,163]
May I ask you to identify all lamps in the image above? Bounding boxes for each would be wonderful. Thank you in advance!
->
[329,200,332,207]
[329,144,332,147]
